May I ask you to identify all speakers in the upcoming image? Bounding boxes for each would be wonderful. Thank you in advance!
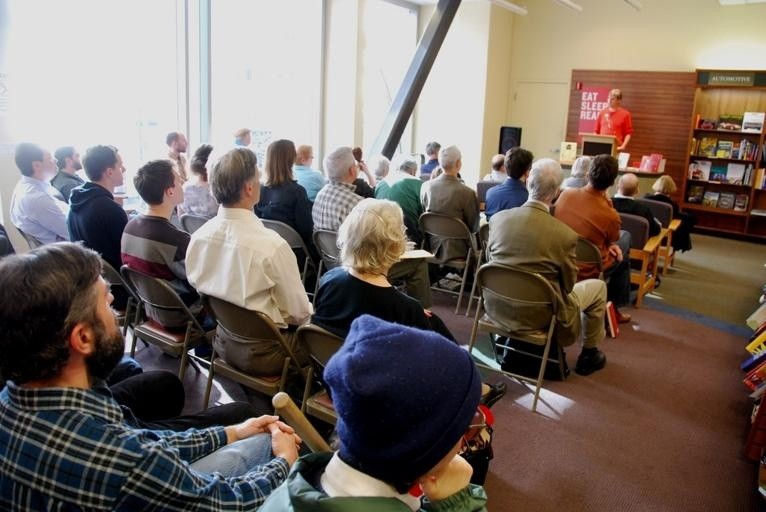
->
[498,126,522,155]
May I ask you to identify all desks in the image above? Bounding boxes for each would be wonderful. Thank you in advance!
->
[560,158,664,198]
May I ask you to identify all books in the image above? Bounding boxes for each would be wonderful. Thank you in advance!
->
[685,113,766,213]
[740,284,766,497]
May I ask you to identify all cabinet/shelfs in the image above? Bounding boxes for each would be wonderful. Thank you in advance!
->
[682,85,766,238]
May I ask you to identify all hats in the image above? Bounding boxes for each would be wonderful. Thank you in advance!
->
[325,315,482,497]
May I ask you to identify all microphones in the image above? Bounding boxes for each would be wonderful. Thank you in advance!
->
[604,114,611,128]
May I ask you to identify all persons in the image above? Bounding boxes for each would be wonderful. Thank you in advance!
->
[553,155,633,323]
[593,89,634,153]
[643,175,680,220]
[0,242,301,512]
[485,160,608,375]
[257,315,489,512]
[610,174,662,288]
[310,142,534,411]
[558,156,593,190]
[8,129,325,380]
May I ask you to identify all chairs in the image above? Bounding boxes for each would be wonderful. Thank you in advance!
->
[469,262,564,412]
[312,230,338,304]
[180,214,207,233]
[638,199,682,276]
[200,294,306,414]
[466,224,490,316]
[295,323,345,426]
[476,181,503,212]
[577,238,603,279]
[259,219,318,285]
[418,212,473,313]
[120,264,215,381]
[15,228,43,251]
[618,213,669,307]
[101,259,150,347]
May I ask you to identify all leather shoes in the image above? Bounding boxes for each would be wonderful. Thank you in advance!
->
[617,313,631,322]
[575,351,606,376]
[481,383,507,407]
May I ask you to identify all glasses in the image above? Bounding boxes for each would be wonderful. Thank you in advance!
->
[464,409,488,446]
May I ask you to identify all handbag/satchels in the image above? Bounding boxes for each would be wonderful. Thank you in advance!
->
[501,337,570,380]
